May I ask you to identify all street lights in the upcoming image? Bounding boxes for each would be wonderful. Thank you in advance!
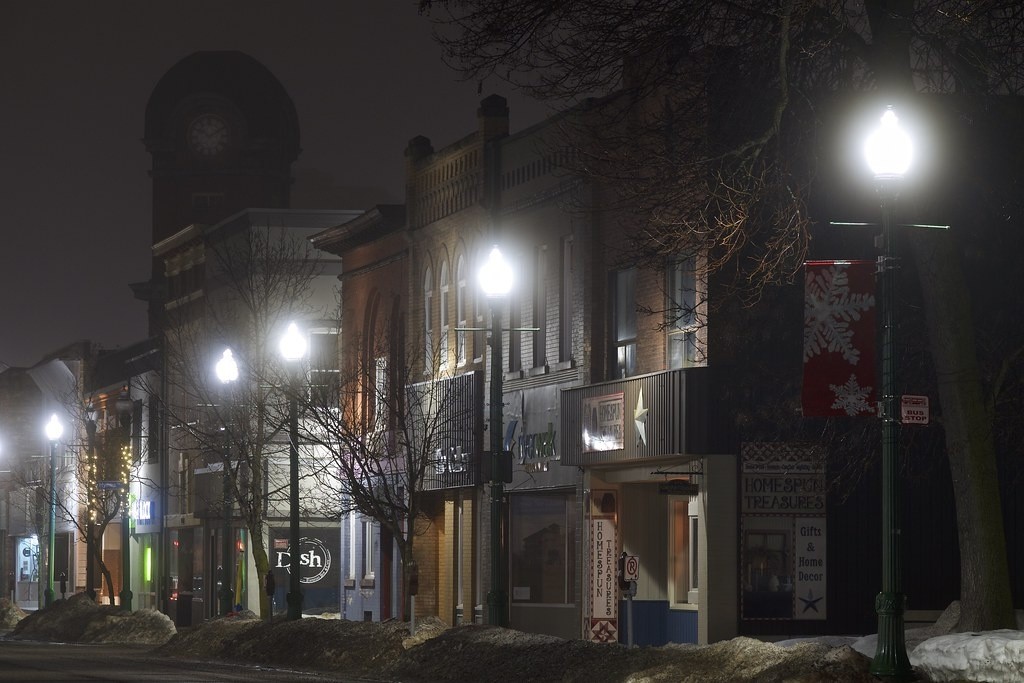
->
[828,105,950,683]
[84,401,100,602]
[452,244,540,628]
[214,346,237,617]
[264,320,340,620]
[30,409,76,611]
